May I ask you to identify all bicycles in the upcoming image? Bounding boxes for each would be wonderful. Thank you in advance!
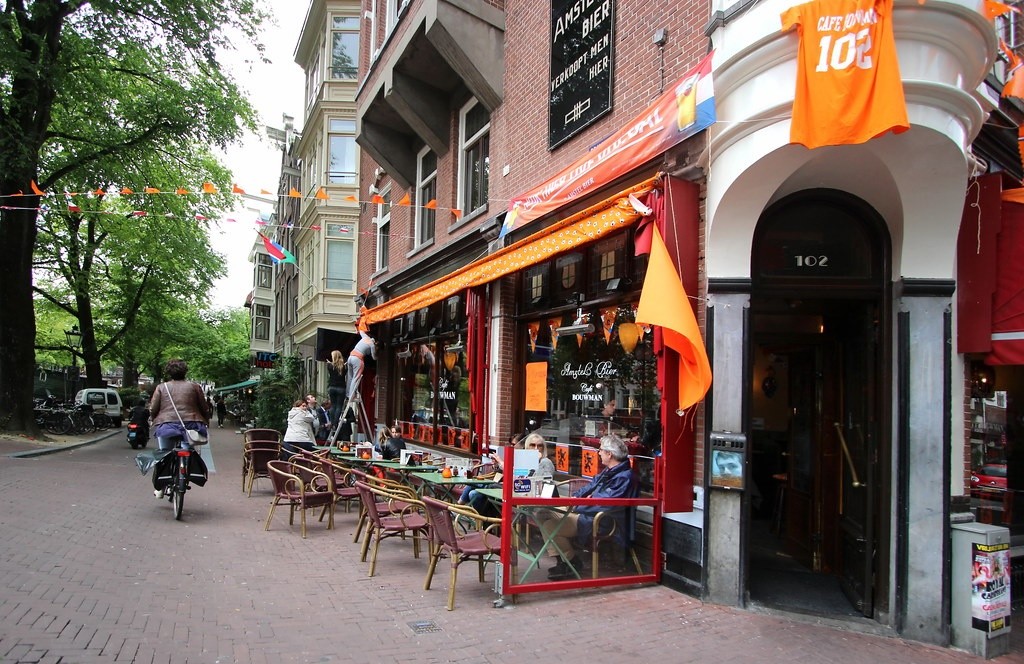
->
[33,395,113,435]
[157,435,192,520]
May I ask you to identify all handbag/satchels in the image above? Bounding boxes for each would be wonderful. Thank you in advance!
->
[186,430,208,446]
[185,447,208,487]
[135,449,175,491]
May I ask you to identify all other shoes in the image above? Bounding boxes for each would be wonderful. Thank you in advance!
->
[221,424,224,427]
[547,555,584,581]
[218,426,220,428]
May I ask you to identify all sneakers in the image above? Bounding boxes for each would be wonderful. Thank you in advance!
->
[154,490,164,499]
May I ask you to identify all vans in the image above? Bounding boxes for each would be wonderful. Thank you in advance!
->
[75,388,125,428]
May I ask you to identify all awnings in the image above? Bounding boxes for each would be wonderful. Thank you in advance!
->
[213,379,257,395]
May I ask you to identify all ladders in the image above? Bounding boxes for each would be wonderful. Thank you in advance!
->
[415,382,455,437]
[323,374,373,447]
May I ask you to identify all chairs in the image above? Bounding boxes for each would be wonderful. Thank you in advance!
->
[422,494,520,612]
[557,467,646,589]
[240,426,434,576]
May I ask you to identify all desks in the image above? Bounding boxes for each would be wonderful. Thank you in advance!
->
[312,441,588,587]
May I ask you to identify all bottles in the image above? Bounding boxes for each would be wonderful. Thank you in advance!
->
[443,464,474,479]
[535,480,560,497]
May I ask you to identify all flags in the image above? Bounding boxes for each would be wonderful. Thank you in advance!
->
[255,228,297,266]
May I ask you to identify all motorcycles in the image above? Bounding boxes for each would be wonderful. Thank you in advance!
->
[127,417,150,450]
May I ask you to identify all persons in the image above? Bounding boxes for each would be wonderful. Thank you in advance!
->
[592,397,622,425]
[372,425,406,487]
[213,394,221,411]
[632,399,661,450]
[204,397,213,428]
[346,329,379,401]
[150,358,212,499]
[217,399,227,428]
[458,433,555,559]
[129,400,150,443]
[713,451,742,478]
[420,344,462,414]
[325,351,348,439]
[454,407,464,428]
[446,433,527,504]
[535,435,641,582]
[281,394,332,462]
